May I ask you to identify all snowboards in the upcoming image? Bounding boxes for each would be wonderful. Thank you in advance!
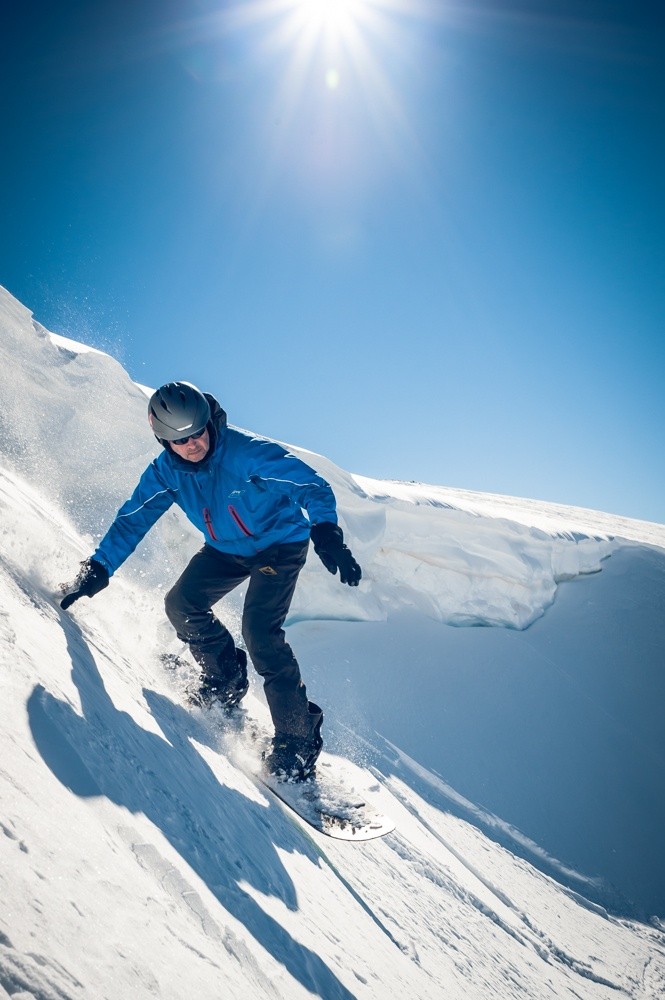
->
[154,651,397,842]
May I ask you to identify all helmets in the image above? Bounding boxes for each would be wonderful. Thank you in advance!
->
[148,381,212,443]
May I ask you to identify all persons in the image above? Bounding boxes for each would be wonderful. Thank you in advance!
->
[60,381,359,788]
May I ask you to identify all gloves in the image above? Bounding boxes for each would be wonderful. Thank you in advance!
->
[60,559,109,610]
[310,521,361,586]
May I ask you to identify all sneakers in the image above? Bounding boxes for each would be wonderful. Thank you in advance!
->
[199,665,249,714]
[264,734,323,783]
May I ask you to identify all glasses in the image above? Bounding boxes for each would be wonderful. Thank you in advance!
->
[171,427,206,445]
